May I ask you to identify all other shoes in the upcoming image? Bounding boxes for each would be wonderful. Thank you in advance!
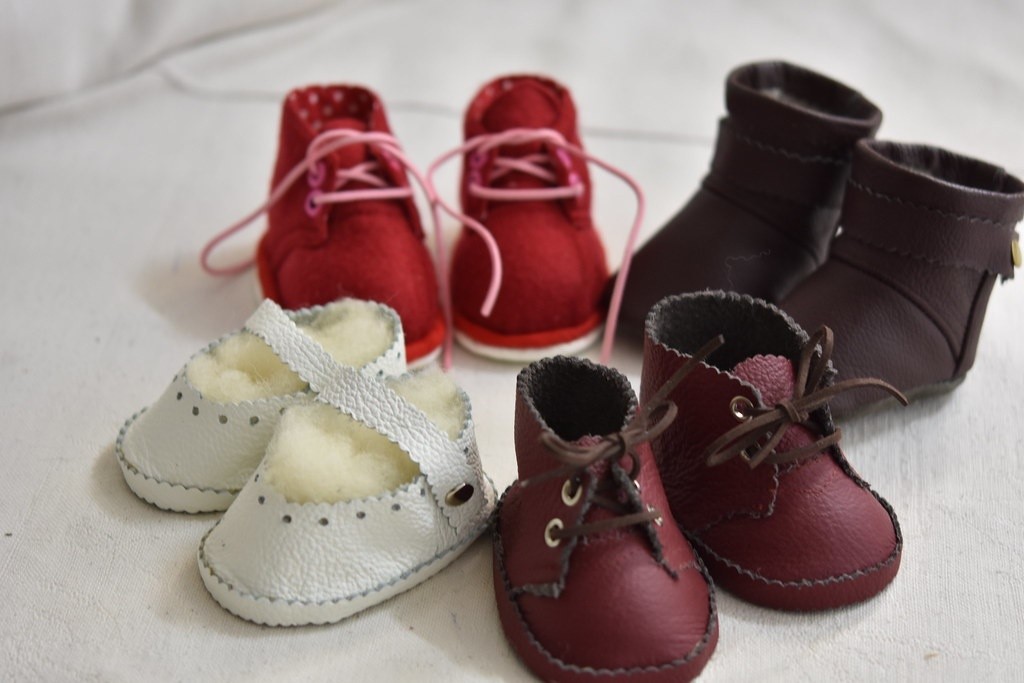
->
[198,370,497,623]
[116,296,407,513]
[782,135,1024,418]
[202,85,445,369]
[428,73,645,362]
[491,356,720,683]
[603,59,882,340]
[643,290,907,611]
